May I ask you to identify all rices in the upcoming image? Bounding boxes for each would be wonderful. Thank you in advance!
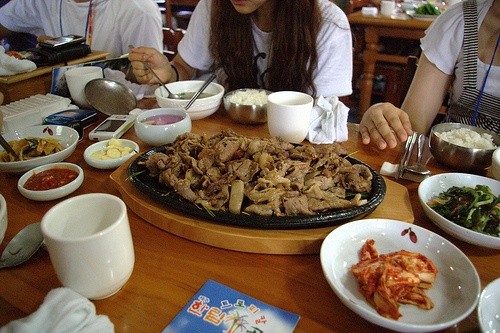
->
[224,89,272,105]
[434,128,497,149]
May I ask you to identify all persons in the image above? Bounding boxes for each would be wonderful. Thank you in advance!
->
[359,0,500,151]
[0,0,163,82]
[128,0,354,106]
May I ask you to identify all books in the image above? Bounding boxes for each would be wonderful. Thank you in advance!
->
[161,278,301,333]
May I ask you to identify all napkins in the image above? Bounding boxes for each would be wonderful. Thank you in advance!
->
[104,67,149,100]
[0,286,115,333]
[306,95,351,145]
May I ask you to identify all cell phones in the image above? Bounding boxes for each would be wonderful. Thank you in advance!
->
[42,34,86,49]
[88,115,135,141]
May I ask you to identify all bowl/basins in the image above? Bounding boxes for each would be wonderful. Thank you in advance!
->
[429,122,500,174]
[134,106,192,146]
[223,88,273,123]
[400,2,417,11]
[154,80,225,121]
[0,124,79,173]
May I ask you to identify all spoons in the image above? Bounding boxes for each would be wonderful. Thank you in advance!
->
[0,222,43,268]
[128,45,182,99]
[404,134,431,175]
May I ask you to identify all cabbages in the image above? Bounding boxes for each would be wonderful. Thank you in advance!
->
[351,240,437,320]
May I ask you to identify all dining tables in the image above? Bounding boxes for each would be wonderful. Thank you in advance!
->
[0,97,500,333]
[345,0,459,124]
[0,51,111,107]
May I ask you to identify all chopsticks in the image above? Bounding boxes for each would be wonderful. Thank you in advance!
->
[0,134,17,157]
[395,132,417,179]
[184,74,217,111]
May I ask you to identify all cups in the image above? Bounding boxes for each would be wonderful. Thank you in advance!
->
[362,7,378,16]
[40,193,135,301]
[267,92,325,144]
[65,66,104,109]
[0,193,8,246]
[492,147,500,181]
[381,0,395,16]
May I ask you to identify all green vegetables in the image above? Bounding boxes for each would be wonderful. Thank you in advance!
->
[413,1,440,16]
[429,183,500,239]
[23,137,39,154]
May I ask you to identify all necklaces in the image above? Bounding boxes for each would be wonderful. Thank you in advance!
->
[59,0,93,49]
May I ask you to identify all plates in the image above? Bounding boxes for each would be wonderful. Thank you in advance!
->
[83,139,139,169]
[127,141,387,229]
[418,172,500,250]
[319,218,500,333]
[405,9,436,21]
[17,162,84,201]
